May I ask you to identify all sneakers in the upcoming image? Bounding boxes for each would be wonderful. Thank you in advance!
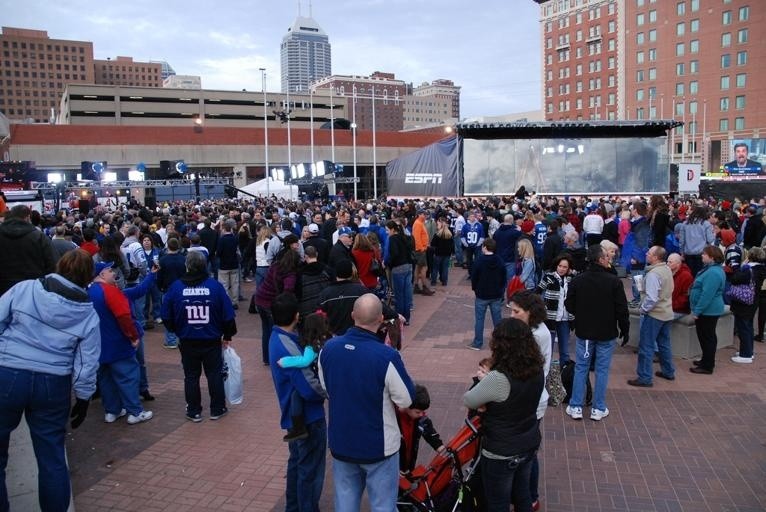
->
[185,410,202,422]
[127,409,154,425]
[729,354,754,365]
[590,407,610,421]
[104,408,126,422]
[465,343,481,351]
[210,406,228,419]
[161,332,178,349]
[565,405,583,419]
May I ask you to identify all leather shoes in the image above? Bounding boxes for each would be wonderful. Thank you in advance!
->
[422,284,436,296]
[413,284,424,294]
[692,360,703,366]
[688,364,714,375]
[626,378,653,388]
[655,371,675,380]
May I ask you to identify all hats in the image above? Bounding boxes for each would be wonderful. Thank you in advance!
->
[416,209,426,214]
[338,226,355,235]
[308,223,319,233]
[93,261,115,275]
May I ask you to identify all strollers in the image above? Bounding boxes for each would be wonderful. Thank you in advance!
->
[399,416,484,512]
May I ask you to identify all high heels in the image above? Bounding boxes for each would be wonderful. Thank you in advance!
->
[140,390,155,402]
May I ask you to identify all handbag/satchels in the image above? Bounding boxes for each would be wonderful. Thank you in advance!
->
[727,264,756,306]
[223,346,245,406]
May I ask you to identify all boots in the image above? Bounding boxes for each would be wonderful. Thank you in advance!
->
[280,416,309,441]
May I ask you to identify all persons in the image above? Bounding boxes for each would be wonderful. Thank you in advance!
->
[515,219,538,261]
[414,186,584,209]
[583,205,604,245]
[138,234,164,324]
[724,143,762,177]
[690,247,726,374]
[744,206,766,251]
[269,295,327,512]
[92,237,130,290]
[464,318,545,512]
[412,210,435,296]
[460,212,485,280]
[215,223,239,309]
[303,224,329,263]
[52,227,76,255]
[186,235,210,263]
[266,218,305,266]
[351,233,381,289]
[713,221,728,255]
[1,248,102,512]
[728,246,766,363]
[543,219,561,268]
[720,229,742,270]
[628,247,676,387]
[59,194,411,222]
[382,221,415,326]
[533,215,548,258]
[298,226,309,262]
[588,192,650,204]
[493,214,521,305]
[679,208,714,276]
[470,357,496,391]
[119,226,155,330]
[666,253,694,313]
[535,254,580,366]
[96,222,114,246]
[509,291,552,509]
[158,238,186,349]
[648,194,670,248]
[600,239,619,276]
[255,226,272,290]
[465,239,507,351]
[453,209,466,267]
[396,385,447,478]
[295,246,328,312]
[670,190,760,207]
[564,244,631,420]
[255,249,300,366]
[431,217,453,286]
[238,213,256,282]
[328,227,357,279]
[512,239,537,292]
[0,211,11,226]
[86,261,153,424]
[277,314,336,441]
[617,211,634,280]
[666,223,684,254]
[620,203,649,308]
[159,251,237,422]
[276,236,301,251]
[604,210,619,244]
[112,266,159,400]
[0,206,60,294]
[562,231,586,267]
[198,220,218,280]
[319,292,415,512]
[80,229,101,256]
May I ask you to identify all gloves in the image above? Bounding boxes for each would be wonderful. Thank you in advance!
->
[70,397,90,430]
[618,331,630,348]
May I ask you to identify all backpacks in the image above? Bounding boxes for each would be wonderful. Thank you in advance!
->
[535,261,547,294]
[562,361,592,404]
